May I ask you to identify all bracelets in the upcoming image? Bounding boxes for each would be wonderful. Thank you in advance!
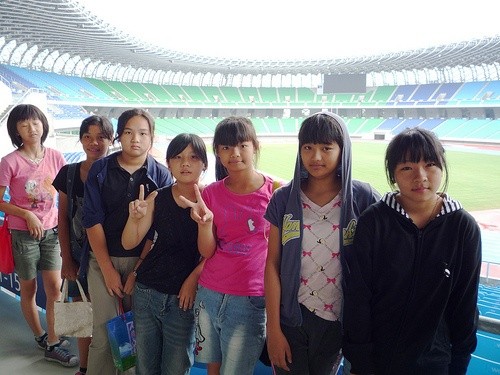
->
[133,267,137,276]
[139,257,144,261]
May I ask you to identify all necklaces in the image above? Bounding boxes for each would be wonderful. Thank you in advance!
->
[398,195,437,230]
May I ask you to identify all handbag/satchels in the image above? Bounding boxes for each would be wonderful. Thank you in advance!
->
[0,211,15,273]
[53,277,94,337]
[106,294,138,372]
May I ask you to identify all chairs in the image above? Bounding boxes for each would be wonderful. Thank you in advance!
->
[0,63,500,144]
[466,280,500,375]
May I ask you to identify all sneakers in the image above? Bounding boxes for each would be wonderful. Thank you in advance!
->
[45,346,78,367]
[35,332,69,349]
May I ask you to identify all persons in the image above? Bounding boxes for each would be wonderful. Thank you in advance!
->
[350,127,482,375]
[52,108,381,375]
[0,104,81,367]
